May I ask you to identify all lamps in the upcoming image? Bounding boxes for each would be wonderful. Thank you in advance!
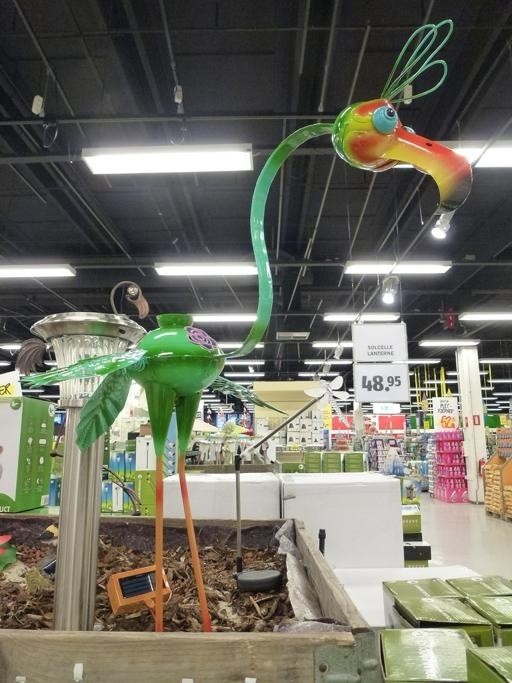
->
[0,342,60,398]
[403,85,413,105]
[31,95,44,116]
[0,264,78,278]
[344,259,454,274]
[431,212,454,238]
[81,142,254,175]
[392,139,512,168]
[174,84,184,103]
[383,275,399,305]
[154,262,260,276]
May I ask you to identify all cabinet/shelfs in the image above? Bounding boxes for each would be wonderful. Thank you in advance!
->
[369,428,512,521]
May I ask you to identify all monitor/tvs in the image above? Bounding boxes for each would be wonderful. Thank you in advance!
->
[55,413,63,425]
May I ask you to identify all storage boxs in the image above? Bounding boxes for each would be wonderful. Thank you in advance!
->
[380,475,512,683]
[0,370,156,517]
[256,408,369,472]
